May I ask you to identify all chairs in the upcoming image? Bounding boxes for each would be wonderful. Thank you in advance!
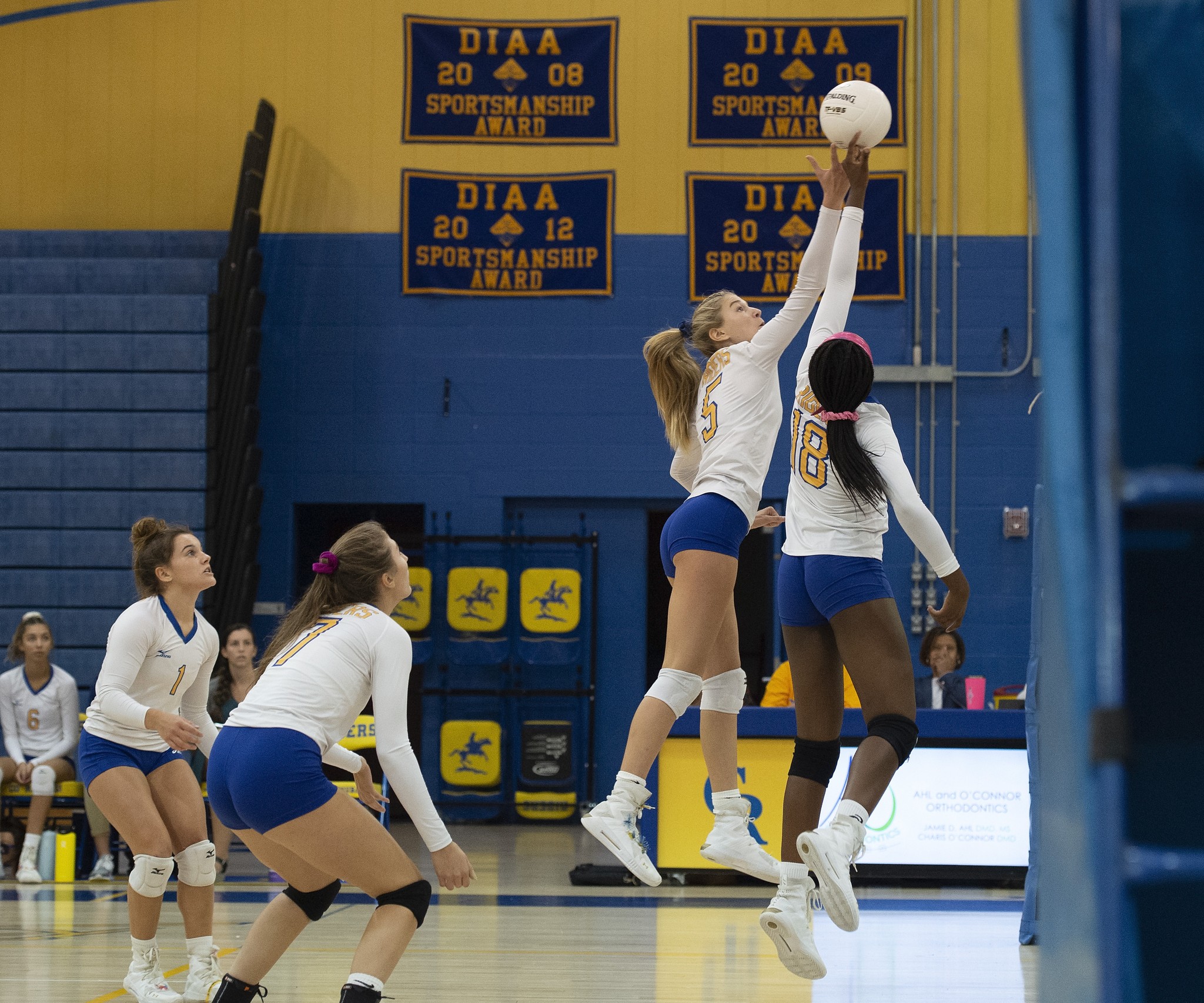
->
[0,704,89,878]
[324,704,394,839]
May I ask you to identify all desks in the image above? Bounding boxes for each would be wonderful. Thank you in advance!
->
[648,690,1041,916]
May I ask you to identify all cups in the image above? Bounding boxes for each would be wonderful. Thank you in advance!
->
[54,833,77,881]
[36,830,56,880]
[965,677,985,710]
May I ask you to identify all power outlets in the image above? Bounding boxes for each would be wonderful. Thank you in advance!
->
[1002,507,1029,539]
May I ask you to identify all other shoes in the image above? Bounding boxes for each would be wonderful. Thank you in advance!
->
[15,851,41,883]
[88,853,115,881]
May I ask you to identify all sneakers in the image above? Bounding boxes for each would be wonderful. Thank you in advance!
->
[759,875,827,980]
[701,797,780,883]
[581,780,662,887]
[797,813,867,932]
[123,945,183,1003]
[183,944,222,1000]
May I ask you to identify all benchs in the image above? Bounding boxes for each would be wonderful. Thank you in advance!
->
[0,225,248,811]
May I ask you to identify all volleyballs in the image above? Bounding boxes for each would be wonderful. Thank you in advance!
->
[819,80,892,152]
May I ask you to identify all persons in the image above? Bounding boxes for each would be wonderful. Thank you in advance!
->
[758,132,971,979]
[759,662,862,710]
[77,517,221,1003]
[580,139,851,888]
[0,713,178,882]
[914,625,968,710]
[0,612,78,884]
[206,525,477,1003]
[189,624,258,877]
